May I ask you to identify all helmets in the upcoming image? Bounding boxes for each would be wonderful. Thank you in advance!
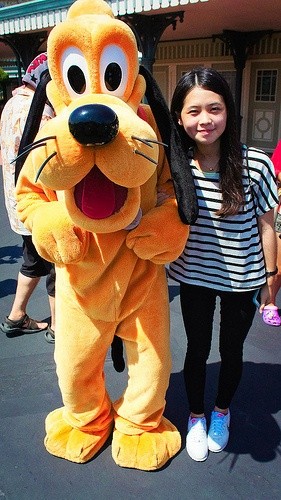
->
[22,52,49,89]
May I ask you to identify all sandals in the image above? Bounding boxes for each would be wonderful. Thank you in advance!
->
[0,312,41,333]
[45,325,55,344]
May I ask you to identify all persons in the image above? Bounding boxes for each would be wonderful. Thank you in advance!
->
[171,67,279,461]
[0,52,56,344]
[263,140,281,325]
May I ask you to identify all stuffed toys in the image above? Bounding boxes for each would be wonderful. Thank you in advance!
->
[15,0,199,471]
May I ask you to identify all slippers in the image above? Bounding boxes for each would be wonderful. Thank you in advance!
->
[262,305,280,326]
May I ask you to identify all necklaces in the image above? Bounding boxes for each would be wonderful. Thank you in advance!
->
[196,155,218,171]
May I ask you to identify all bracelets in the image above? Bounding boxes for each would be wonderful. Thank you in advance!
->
[266,266,278,276]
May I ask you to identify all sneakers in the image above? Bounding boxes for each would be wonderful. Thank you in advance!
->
[207,407,231,453]
[186,415,209,462]
